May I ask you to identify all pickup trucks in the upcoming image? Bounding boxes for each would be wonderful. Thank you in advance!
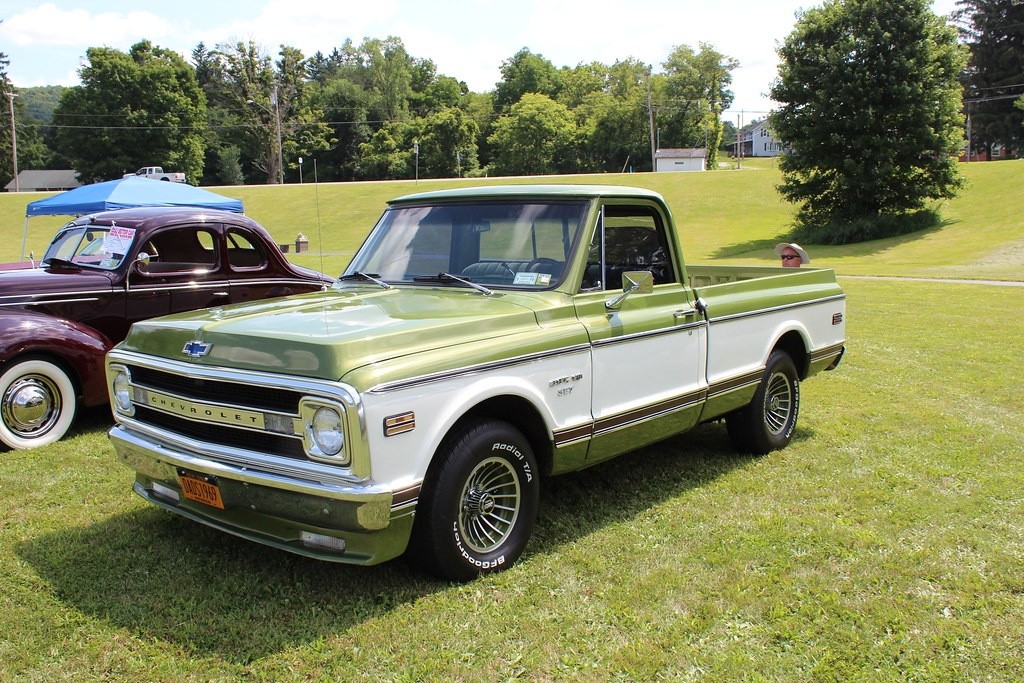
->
[105,182,848,584]
[122,166,187,184]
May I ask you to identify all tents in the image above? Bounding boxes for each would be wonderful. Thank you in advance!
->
[21,176,253,262]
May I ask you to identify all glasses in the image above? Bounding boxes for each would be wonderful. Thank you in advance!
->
[781,255,800,260]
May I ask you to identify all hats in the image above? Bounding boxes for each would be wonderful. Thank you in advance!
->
[773,242,810,265]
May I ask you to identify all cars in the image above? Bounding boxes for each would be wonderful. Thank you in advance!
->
[1,205,336,450]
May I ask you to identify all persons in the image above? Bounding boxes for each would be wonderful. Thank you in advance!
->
[143,169,146,173]
[149,169,152,172]
[774,242,811,267]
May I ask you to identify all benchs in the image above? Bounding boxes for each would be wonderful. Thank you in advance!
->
[462,260,668,292]
[133,261,231,272]
